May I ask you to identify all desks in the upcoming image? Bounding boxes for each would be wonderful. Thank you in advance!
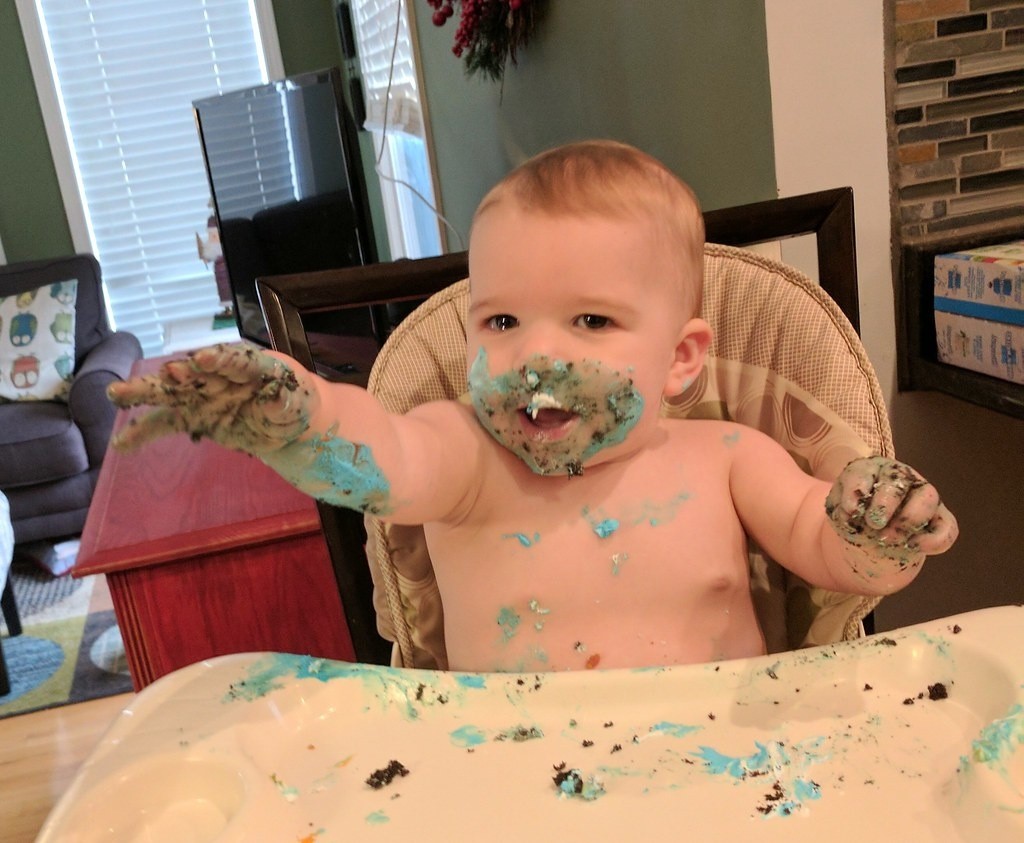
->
[72,353,355,692]
[32,602,1024,843]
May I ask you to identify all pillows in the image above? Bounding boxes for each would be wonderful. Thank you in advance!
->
[0,277,79,402]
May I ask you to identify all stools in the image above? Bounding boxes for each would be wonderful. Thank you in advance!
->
[0,492,24,696]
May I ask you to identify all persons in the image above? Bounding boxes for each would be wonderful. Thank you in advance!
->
[103,140,959,673]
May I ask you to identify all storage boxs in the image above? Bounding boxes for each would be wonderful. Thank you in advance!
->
[934,242,1024,328]
[935,310,1024,385]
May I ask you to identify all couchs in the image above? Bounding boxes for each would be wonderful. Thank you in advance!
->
[1,253,144,552]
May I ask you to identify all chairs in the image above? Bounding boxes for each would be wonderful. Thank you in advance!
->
[255,185,877,666]
